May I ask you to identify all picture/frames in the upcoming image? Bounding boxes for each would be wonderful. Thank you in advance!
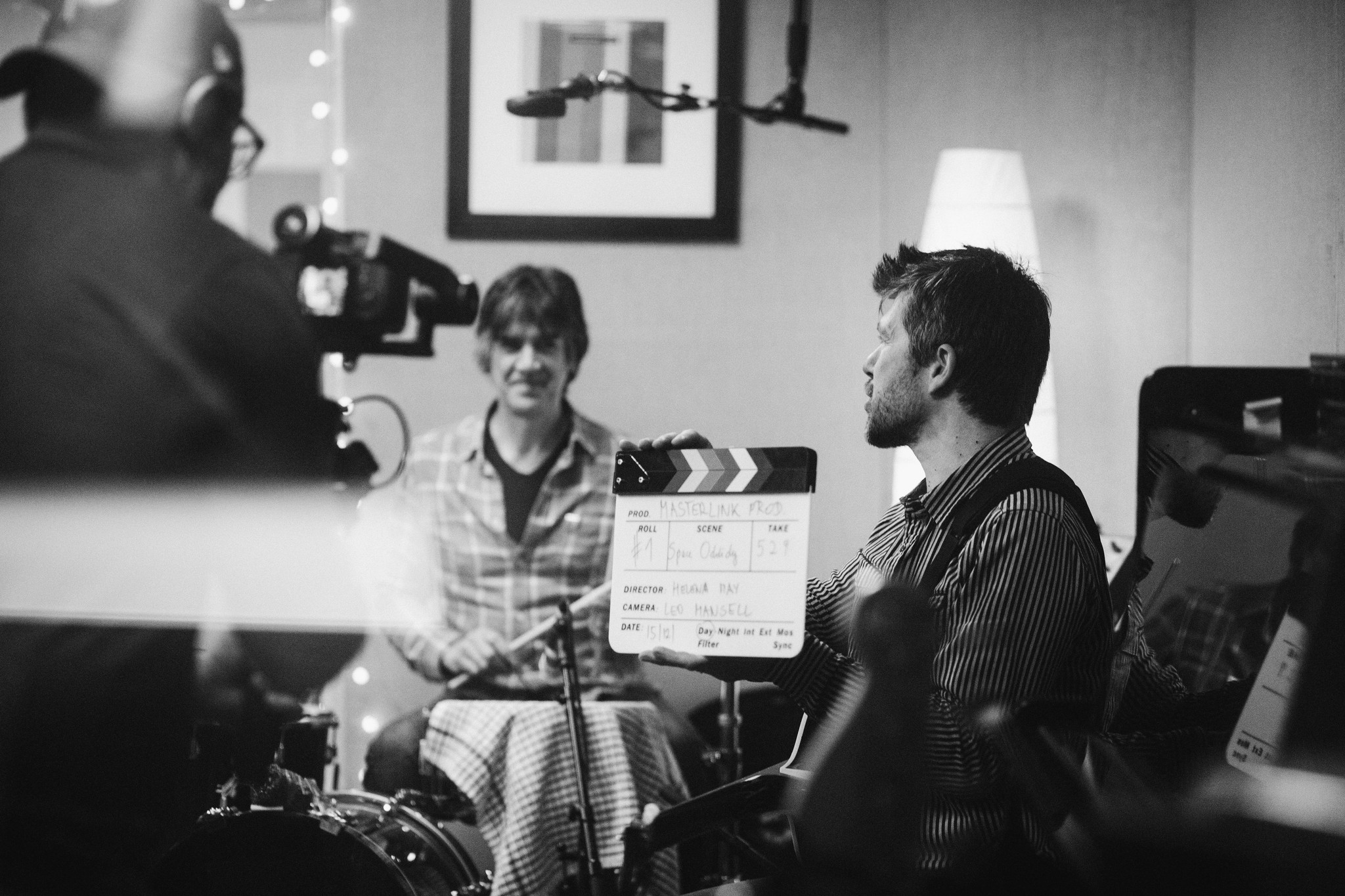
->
[445,0,745,245]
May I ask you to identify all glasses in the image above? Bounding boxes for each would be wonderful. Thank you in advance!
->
[195,76,265,180]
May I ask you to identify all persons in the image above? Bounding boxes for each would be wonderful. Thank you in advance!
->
[0,1,366,895]
[362,266,710,797]
[617,242,1112,895]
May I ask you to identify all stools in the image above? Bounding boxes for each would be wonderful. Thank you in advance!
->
[361,652,721,828]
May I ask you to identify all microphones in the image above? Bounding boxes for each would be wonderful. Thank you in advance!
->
[506,88,567,119]
